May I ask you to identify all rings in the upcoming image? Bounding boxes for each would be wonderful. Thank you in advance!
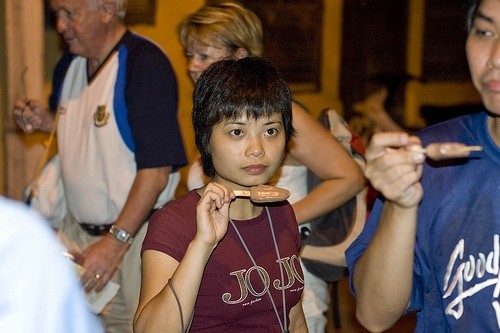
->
[94,273,103,280]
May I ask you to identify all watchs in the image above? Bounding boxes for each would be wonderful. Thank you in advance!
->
[109,223,135,245]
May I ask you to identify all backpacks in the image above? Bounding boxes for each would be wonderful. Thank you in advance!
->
[290,98,379,283]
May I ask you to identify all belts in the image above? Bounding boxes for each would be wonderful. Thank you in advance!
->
[76,208,160,236]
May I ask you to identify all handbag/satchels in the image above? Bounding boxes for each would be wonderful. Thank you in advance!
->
[24,151,68,228]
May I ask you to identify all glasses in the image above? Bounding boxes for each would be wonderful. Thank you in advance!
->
[20,66,34,134]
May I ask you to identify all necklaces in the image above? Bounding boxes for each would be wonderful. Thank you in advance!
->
[228,204,287,332]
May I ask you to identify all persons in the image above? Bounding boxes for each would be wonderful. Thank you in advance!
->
[0,0,500,333]
